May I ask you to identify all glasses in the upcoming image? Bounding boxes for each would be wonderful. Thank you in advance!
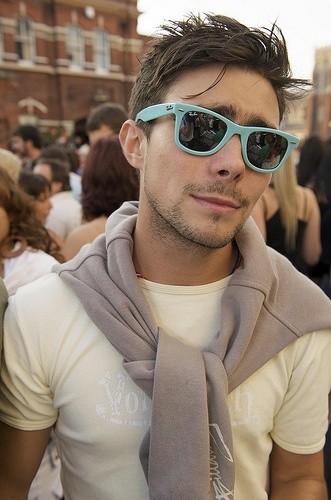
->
[135,103,301,173]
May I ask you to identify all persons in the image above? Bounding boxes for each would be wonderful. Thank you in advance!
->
[0,10,331,500]
[0,166,68,500]
[0,104,331,297]
